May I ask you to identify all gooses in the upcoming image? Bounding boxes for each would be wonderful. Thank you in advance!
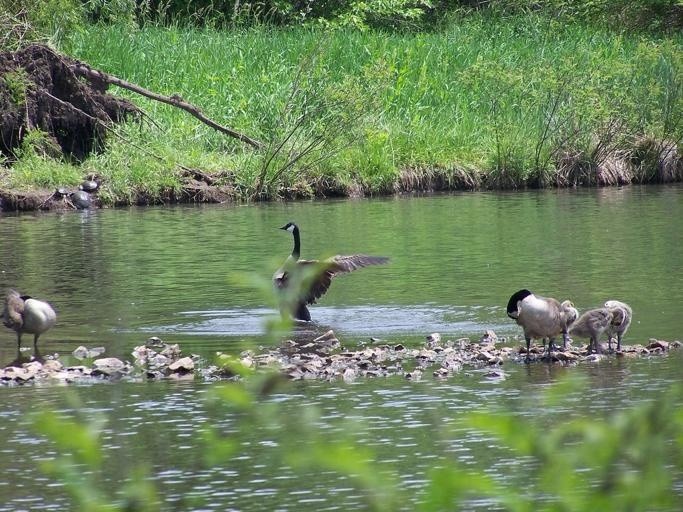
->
[0,288,56,368]
[506,289,633,362]
[272,222,392,322]
